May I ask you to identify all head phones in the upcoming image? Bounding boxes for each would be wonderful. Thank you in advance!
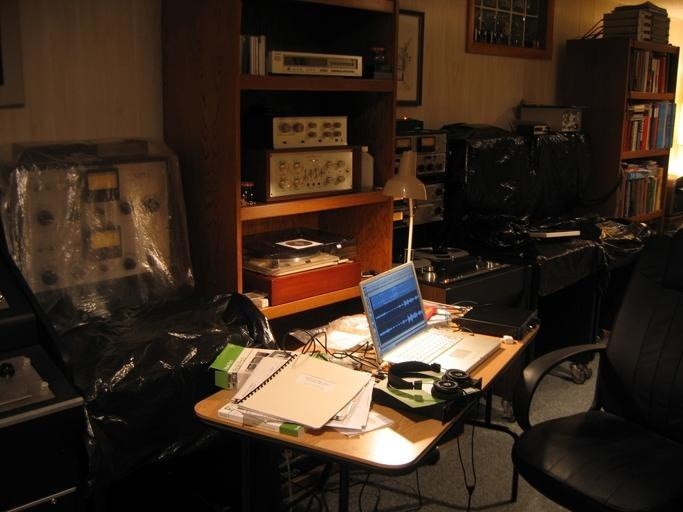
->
[388,361,482,400]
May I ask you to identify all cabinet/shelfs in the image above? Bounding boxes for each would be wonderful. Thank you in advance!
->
[562,38,679,234]
[160,2,400,349]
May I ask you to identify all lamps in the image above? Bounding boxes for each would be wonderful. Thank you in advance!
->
[381,150,432,271]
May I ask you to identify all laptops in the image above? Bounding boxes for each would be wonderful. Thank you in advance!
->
[359,261,501,375]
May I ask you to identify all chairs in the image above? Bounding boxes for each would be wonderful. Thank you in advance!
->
[511,235,683,511]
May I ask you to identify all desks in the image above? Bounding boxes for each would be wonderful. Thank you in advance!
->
[194,298,542,510]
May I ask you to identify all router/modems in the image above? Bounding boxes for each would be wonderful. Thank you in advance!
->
[457,303,542,340]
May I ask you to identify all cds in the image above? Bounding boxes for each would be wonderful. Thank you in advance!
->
[286,241,312,246]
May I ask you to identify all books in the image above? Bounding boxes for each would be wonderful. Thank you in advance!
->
[622,48,676,218]
[242,34,266,77]
[528,230,581,238]
[218,353,394,437]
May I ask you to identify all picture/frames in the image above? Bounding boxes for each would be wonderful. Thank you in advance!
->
[398,8,426,108]
